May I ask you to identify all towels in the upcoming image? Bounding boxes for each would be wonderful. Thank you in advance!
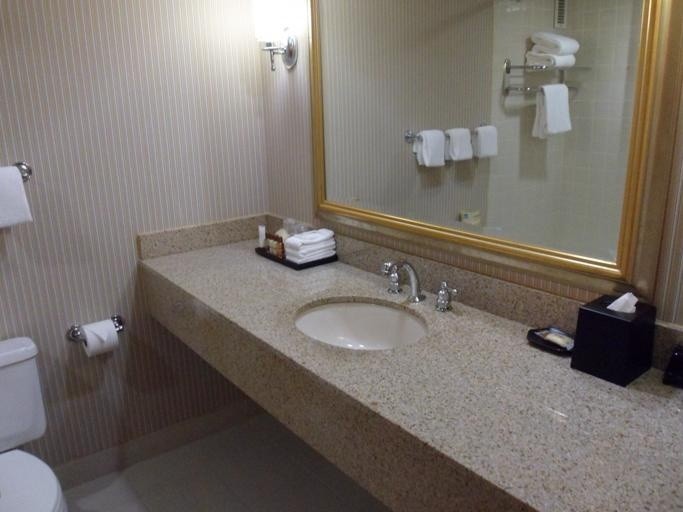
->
[410,125,499,169]
[0,162,33,232]
[532,83,573,140]
[525,31,580,67]
[283,228,336,264]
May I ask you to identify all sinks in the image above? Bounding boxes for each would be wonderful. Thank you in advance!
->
[294,296,428,350]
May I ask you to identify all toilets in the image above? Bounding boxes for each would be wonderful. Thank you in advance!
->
[0,337,68,512]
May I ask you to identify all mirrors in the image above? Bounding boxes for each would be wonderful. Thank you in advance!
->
[307,0,682,307]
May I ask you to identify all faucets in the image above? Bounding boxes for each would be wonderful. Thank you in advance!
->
[382,259,422,302]
